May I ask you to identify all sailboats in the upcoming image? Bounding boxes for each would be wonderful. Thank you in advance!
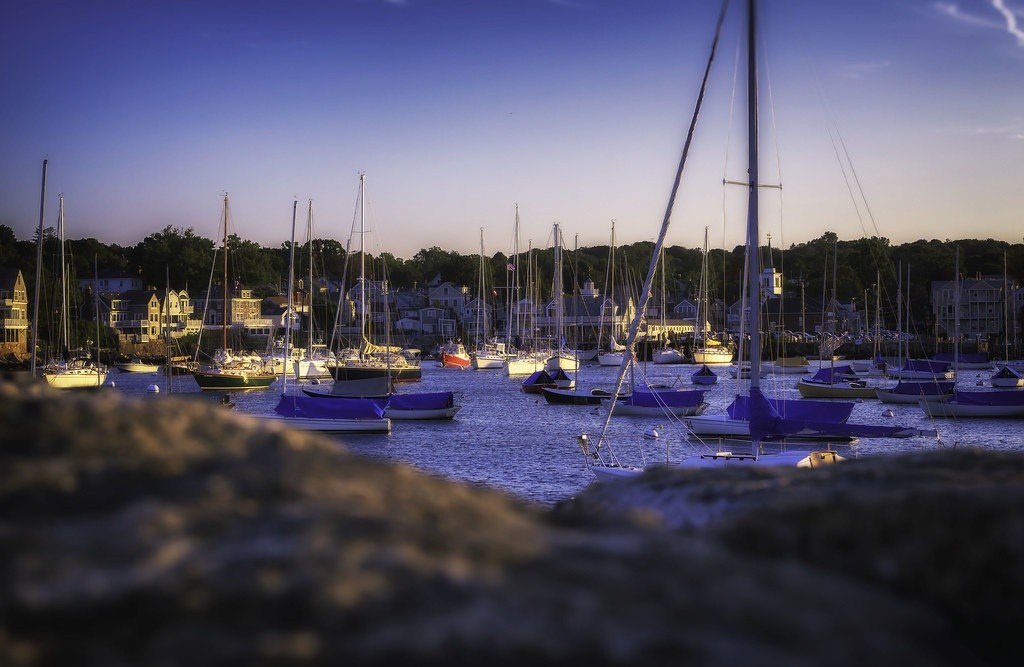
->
[474,225,506,369]
[989,250,1024,388]
[293,200,347,380]
[580,0,850,487]
[502,205,959,416]
[237,200,392,435]
[274,200,462,421]
[164,288,200,377]
[324,175,423,384]
[683,209,939,446]
[42,193,109,389]
[191,192,278,391]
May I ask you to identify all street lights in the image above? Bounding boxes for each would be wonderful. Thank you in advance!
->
[864,288,872,338]
[871,283,876,337]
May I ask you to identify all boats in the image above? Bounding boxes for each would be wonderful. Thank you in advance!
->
[917,387,1024,419]
[210,342,307,376]
[439,337,471,370]
[111,359,159,373]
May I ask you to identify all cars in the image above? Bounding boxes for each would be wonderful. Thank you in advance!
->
[640,329,924,344]
